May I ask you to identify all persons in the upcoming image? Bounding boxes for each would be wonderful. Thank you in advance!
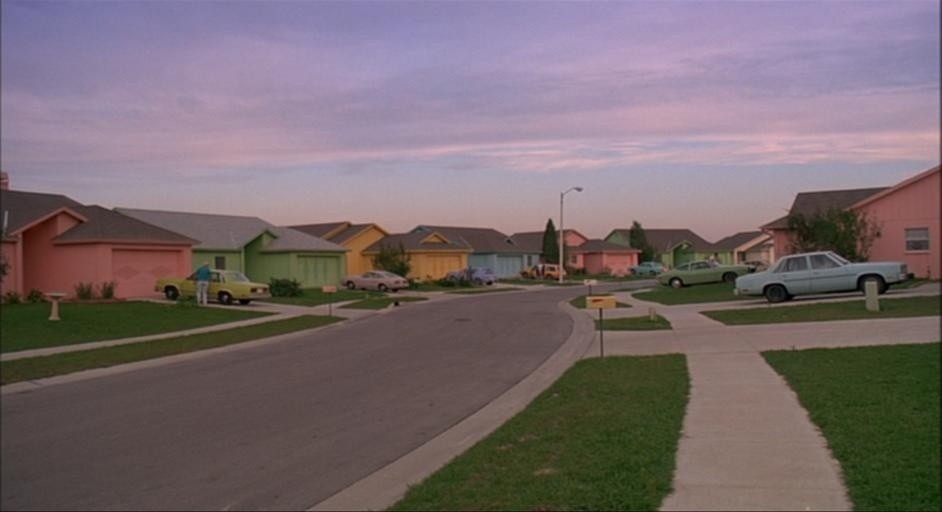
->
[196,261,212,306]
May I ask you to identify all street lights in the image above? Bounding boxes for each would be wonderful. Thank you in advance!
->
[558,185,583,283]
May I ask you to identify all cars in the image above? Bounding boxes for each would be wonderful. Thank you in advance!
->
[733,250,910,303]
[658,258,758,289]
[340,270,410,292]
[629,261,663,276]
[445,266,495,286]
[154,269,272,306]
[741,259,769,273]
[521,263,567,280]
